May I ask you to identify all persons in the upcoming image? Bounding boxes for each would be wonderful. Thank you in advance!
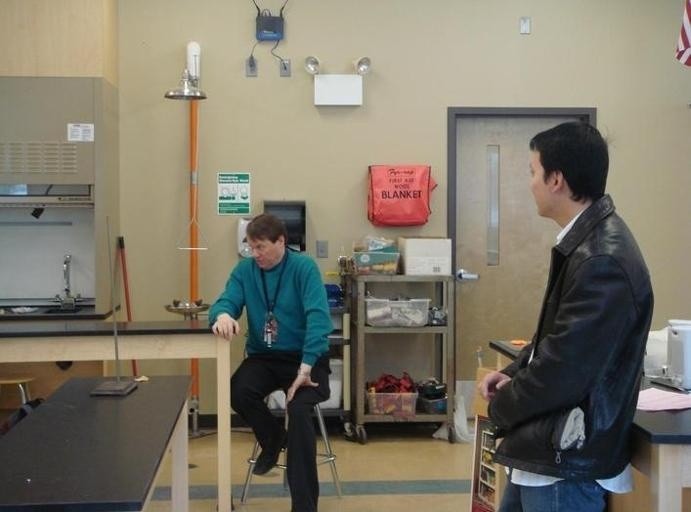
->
[207,212,334,512]
[476,118,656,511]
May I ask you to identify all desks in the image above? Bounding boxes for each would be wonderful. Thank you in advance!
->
[0,375,194,512]
[0,319,236,511]
[470,340,691,512]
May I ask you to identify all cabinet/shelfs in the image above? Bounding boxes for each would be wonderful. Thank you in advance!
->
[0,76,120,314]
[342,274,459,445]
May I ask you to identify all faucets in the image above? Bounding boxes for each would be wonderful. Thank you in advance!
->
[62,254,72,298]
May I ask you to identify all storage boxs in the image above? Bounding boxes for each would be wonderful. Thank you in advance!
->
[397,236,452,276]
[314,357,343,409]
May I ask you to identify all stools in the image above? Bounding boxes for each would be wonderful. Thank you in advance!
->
[241,387,343,503]
[0,375,37,406]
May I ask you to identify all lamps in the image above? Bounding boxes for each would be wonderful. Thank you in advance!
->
[354,55,372,77]
[165,41,216,440]
[303,55,320,76]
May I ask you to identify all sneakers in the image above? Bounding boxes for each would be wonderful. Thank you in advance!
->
[251,428,286,475]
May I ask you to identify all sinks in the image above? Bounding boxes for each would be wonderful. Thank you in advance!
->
[44,308,83,314]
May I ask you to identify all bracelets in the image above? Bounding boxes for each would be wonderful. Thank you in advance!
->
[296,368,312,377]
